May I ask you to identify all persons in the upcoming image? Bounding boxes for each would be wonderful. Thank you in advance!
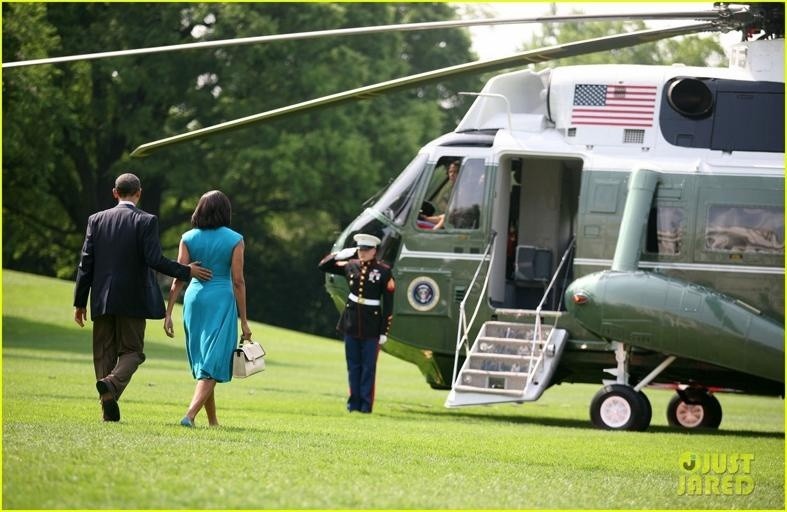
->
[164,189,254,429]
[316,232,396,414]
[71,171,214,421]
[421,159,462,231]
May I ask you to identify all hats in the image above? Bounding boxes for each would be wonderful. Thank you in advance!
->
[353,233,383,249]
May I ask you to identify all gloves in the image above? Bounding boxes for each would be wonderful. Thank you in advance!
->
[379,334,388,345]
[336,248,357,261]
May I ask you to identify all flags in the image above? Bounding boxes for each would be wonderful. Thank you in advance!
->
[571,82,659,129]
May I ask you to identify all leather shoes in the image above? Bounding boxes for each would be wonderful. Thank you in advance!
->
[96,377,121,421]
[180,416,195,428]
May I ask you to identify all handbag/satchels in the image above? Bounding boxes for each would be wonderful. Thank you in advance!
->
[232,337,267,379]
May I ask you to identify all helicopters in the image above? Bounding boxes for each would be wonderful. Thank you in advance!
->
[2,3,784,433]
